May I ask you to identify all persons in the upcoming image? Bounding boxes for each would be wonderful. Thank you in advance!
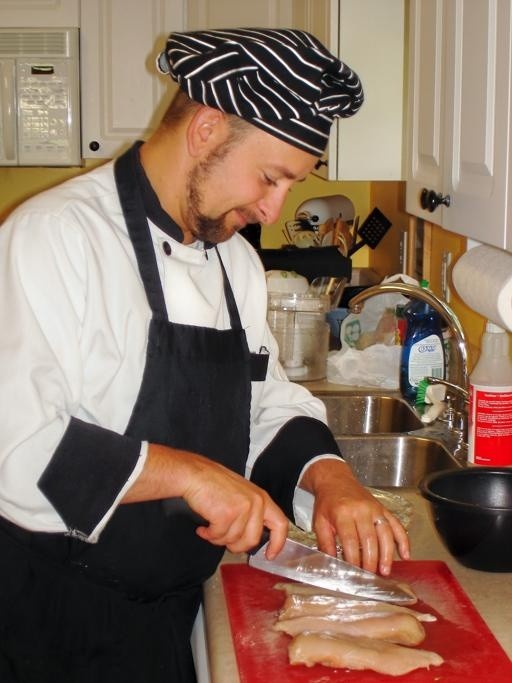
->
[0,26,411,683]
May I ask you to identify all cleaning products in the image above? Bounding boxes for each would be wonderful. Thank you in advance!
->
[402,278,446,400]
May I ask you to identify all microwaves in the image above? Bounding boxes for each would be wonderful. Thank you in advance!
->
[1,28,85,168]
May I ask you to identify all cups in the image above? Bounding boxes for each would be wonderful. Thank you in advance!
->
[310,277,347,309]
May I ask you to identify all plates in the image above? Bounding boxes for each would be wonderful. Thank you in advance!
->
[302,486,414,543]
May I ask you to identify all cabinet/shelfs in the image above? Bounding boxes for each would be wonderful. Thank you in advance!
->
[81,0,405,181]
[405,0,511,254]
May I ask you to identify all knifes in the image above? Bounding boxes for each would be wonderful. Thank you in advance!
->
[248,527,418,609]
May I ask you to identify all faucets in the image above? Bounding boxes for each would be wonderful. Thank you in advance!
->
[346,281,473,433]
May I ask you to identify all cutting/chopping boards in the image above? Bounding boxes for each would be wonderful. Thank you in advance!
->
[220,561,512,683]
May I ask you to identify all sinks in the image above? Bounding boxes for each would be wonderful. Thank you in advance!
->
[306,389,429,435]
[332,434,463,492]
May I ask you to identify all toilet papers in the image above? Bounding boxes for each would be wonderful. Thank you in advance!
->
[451,243,512,332]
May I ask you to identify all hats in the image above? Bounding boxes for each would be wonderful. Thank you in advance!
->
[155,27,364,158]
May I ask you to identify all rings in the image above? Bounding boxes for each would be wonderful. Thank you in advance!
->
[374,519,386,527]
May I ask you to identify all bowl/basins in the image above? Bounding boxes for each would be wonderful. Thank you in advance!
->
[340,282,469,391]
[417,459,511,570]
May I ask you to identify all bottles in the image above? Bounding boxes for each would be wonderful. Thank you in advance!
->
[399,291,451,406]
[267,294,331,382]
[294,193,355,230]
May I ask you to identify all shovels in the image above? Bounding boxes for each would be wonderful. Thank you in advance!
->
[348,207,392,260]
